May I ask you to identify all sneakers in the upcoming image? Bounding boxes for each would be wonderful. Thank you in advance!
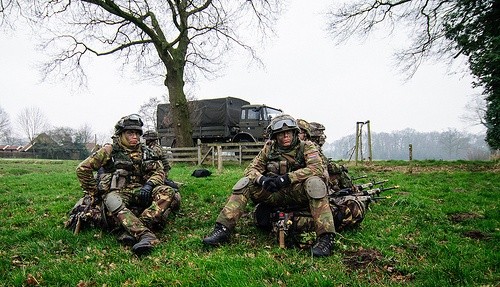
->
[117,233,135,243]
[133,233,160,251]
[313,234,335,257]
[204,226,232,246]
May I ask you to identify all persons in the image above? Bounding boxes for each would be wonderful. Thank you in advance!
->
[202,115,366,258]
[76,114,182,253]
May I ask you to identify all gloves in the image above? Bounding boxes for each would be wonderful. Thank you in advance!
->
[259,175,279,193]
[339,165,348,173]
[139,183,154,203]
[276,174,290,188]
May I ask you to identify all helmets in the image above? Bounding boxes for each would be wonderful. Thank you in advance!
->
[267,115,301,140]
[296,119,311,137]
[143,131,158,140]
[310,122,325,136]
[115,115,143,136]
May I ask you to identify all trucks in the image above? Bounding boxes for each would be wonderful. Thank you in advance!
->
[157,97,283,161]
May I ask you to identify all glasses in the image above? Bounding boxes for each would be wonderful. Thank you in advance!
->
[130,115,144,126]
[271,120,297,131]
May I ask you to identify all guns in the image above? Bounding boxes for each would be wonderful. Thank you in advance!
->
[97,167,115,231]
[351,174,402,202]
